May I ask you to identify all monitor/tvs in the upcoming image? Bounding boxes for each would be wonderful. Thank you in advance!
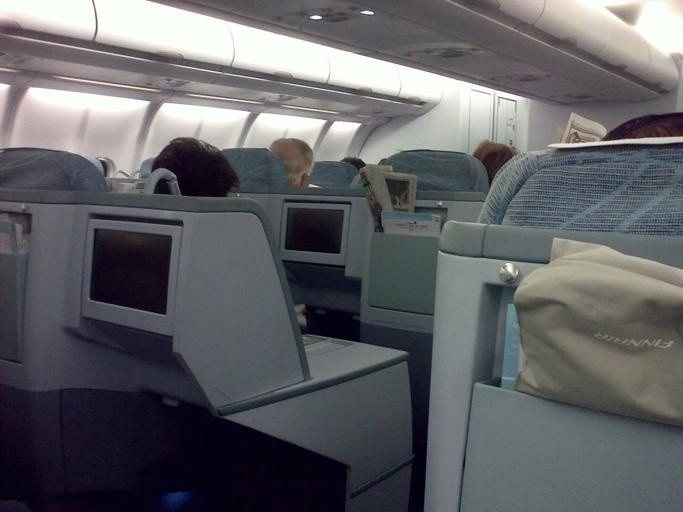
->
[279,203,350,266]
[82,220,182,336]
[414,206,448,233]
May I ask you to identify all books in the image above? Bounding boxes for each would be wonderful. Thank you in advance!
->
[379,209,440,237]
[350,163,394,233]
[371,170,417,213]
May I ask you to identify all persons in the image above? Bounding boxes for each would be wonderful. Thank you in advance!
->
[149,135,241,196]
[340,156,366,171]
[267,137,316,188]
[470,137,522,189]
[597,111,683,141]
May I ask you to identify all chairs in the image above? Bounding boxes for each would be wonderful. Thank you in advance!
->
[0,137,683,480]
